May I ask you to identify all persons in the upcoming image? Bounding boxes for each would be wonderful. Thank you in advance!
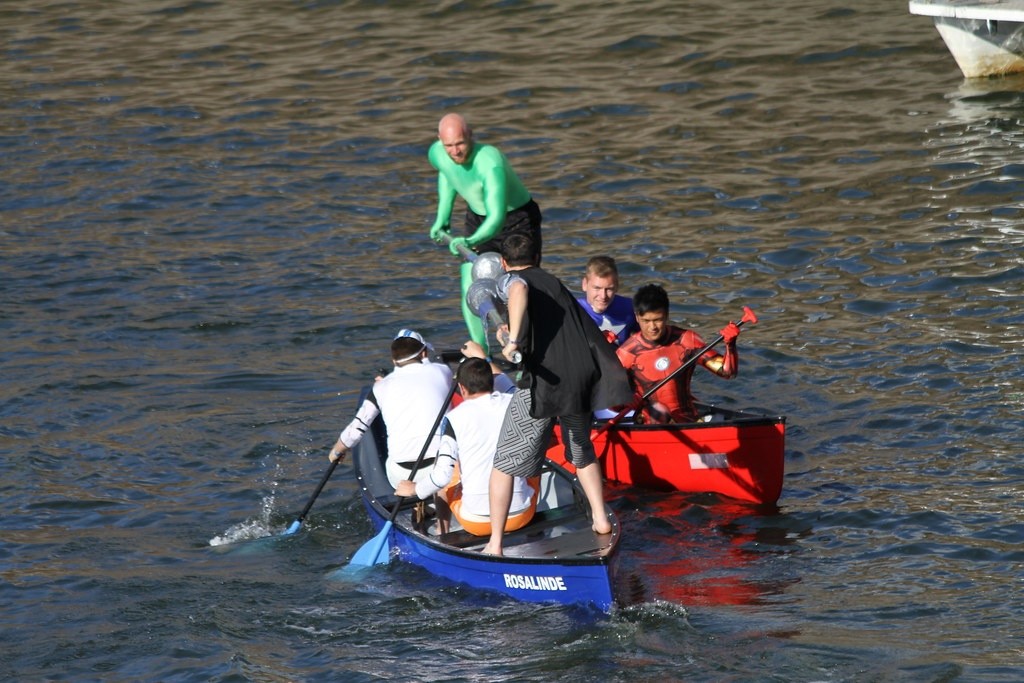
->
[428,114,542,353]
[576,256,641,350]
[328,328,454,488]
[394,341,540,535]
[616,284,740,424]
[482,233,633,555]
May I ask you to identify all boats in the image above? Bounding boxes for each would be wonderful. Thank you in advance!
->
[909,0,1024,79]
[353,385,623,619]
[434,346,786,505]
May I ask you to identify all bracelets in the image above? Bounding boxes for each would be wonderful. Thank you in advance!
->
[509,339,520,344]
[485,357,492,363]
[333,450,340,456]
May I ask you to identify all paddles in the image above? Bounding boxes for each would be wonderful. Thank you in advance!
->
[558,307,757,467]
[281,367,389,535]
[349,343,466,566]
